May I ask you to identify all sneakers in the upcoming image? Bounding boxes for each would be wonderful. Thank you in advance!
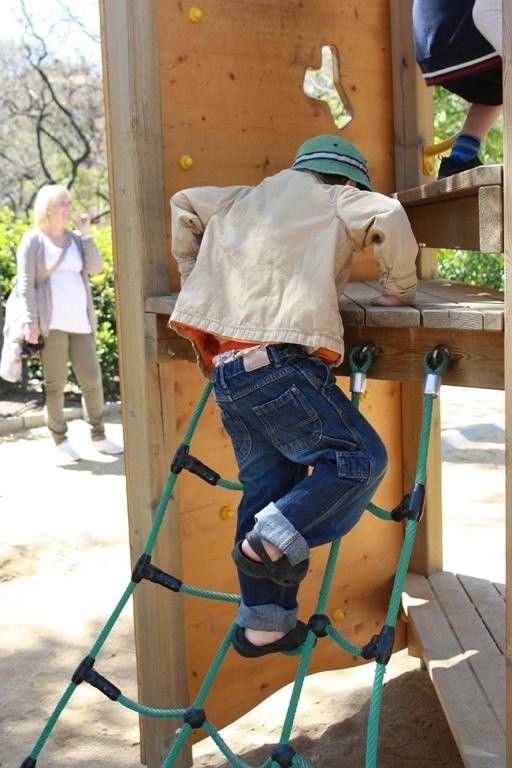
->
[436,151,483,175]
[93,435,123,455]
[52,437,80,463]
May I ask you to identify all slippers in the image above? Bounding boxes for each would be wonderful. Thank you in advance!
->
[229,619,310,658]
[234,529,308,585]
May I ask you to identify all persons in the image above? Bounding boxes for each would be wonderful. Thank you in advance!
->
[16,184,124,461]
[412,0,502,181]
[169,130,421,657]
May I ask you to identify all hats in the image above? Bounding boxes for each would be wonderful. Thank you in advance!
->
[291,134,375,190]
